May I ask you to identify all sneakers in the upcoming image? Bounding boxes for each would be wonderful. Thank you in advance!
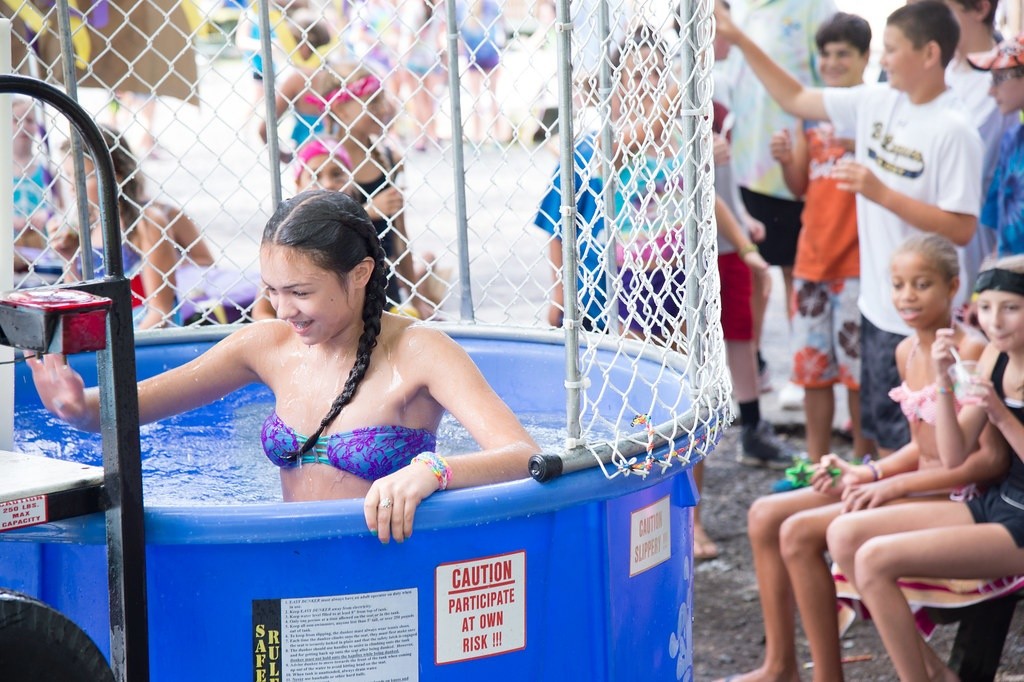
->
[736,421,803,470]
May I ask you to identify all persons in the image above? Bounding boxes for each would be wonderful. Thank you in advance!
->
[12,0,505,322]
[534,2,1024,682]
[23,189,543,544]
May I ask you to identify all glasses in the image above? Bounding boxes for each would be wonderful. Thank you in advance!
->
[992,73,1024,85]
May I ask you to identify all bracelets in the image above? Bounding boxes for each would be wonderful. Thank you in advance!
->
[865,454,882,480]
[411,451,452,491]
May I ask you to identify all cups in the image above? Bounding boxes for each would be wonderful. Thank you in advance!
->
[947,361,984,404]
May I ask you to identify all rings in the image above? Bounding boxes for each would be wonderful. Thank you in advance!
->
[381,498,392,510]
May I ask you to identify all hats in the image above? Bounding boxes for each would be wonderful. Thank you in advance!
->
[967,32,1024,71]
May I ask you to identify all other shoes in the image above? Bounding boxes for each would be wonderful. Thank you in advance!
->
[781,381,805,410]
[760,371,773,393]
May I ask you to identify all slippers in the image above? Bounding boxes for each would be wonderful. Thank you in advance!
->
[693,540,718,561]
